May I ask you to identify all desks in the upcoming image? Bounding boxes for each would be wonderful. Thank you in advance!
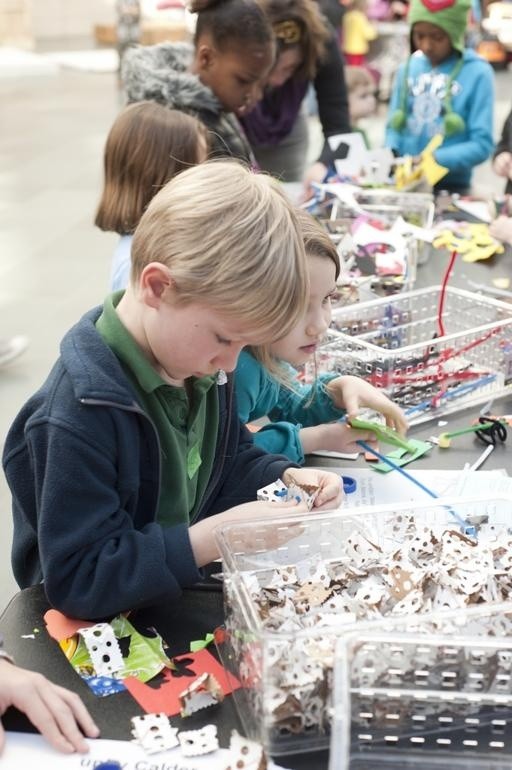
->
[1,235,510,770]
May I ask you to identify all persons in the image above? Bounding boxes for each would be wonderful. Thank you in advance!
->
[1,161,345,620]
[492,100,512,196]
[94,101,209,292]
[343,65,378,151]
[0,637,101,759]
[383,0,495,196]
[343,0,379,65]
[122,0,275,173]
[234,0,351,180]
[233,206,409,465]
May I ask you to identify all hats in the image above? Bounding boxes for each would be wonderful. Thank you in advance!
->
[407,0,471,61]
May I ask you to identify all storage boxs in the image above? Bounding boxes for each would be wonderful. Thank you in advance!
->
[214,489,512,756]
[302,283,512,429]
[329,631,512,770]
